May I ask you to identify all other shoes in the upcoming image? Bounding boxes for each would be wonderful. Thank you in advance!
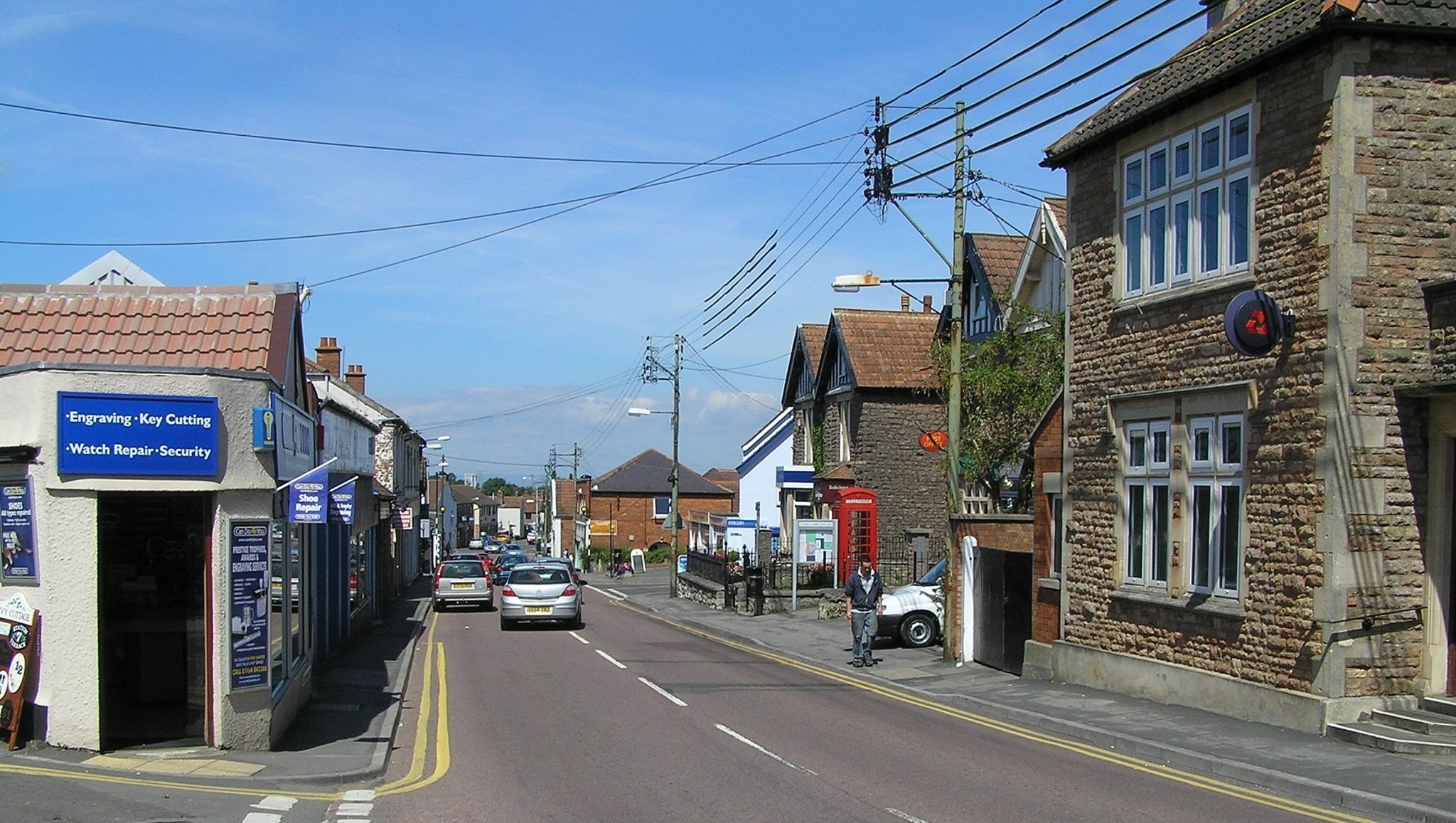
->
[864,658,874,666]
[854,660,864,667]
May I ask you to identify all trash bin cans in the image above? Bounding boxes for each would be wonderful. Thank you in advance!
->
[745,565,764,599]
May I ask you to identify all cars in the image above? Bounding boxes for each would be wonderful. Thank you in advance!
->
[444,553,482,575]
[477,552,496,584]
[525,556,583,604]
[500,562,587,631]
[270,518,368,609]
[876,557,948,648]
[525,523,545,545]
[484,541,504,554]
[491,554,531,586]
[501,544,524,555]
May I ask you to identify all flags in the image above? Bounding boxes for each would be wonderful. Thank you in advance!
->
[327,480,356,524]
[289,464,329,524]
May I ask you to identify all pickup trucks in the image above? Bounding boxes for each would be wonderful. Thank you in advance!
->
[496,534,511,544]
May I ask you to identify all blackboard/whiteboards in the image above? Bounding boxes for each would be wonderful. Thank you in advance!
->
[631,548,646,574]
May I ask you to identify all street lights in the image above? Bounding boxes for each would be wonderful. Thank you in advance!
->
[831,273,966,660]
[525,499,547,555]
[440,504,446,564]
[628,407,680,598]
[522,476,539,556]
[435,472,447,569]
[471,497,480,538]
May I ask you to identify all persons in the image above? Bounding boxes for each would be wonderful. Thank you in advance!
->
[569,553,574,568]
[563,548,569,559]
[844,555,883,667]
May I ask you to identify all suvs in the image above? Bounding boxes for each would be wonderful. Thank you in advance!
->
[469,538,484,550]
[427,560,494,612]
